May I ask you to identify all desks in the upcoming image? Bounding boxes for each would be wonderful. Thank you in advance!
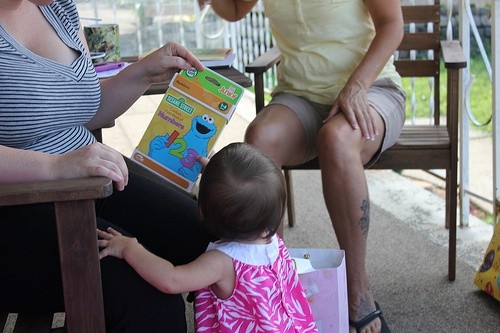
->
[92,56,252,102]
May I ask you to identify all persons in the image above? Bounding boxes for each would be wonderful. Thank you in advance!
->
[96,143,318,333]
[0,0,211,333]
[211,0,406,333]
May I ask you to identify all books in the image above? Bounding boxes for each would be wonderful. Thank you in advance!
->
[139,46,236,69]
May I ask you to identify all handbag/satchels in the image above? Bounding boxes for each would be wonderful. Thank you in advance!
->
[287,247,351,333]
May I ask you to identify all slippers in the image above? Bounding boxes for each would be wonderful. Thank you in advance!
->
[349,300,390,333]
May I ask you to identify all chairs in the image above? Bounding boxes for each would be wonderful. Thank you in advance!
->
[245,1,468,286]
[0,116,121,333]
